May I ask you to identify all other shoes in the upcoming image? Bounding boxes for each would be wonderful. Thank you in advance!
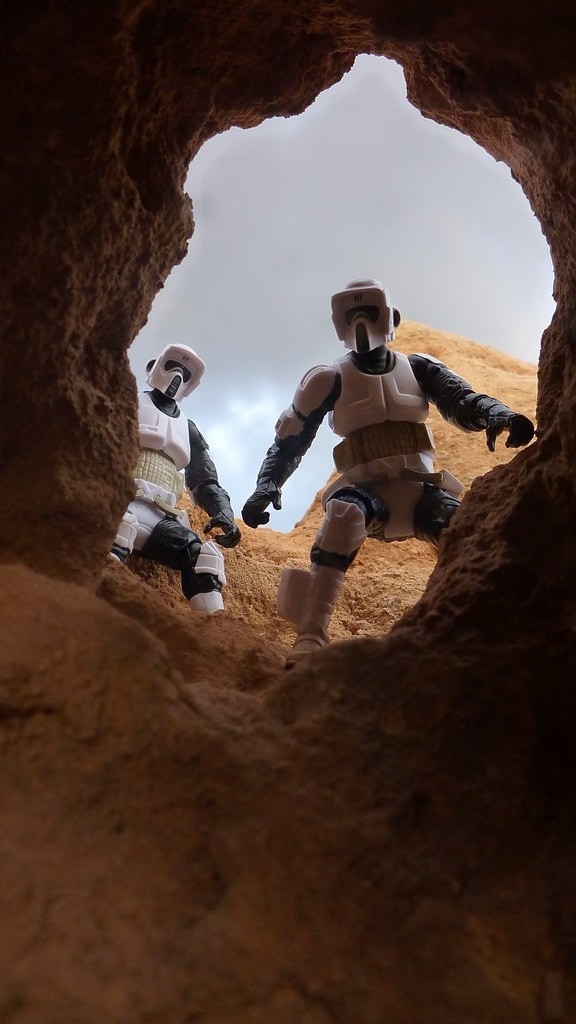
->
[286,633,328,671]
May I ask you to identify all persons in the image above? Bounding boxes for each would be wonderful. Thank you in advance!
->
[241,276,535,670]
[109,343,241,614]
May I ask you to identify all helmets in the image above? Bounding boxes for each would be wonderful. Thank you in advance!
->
[145,344,207,402]
[331,277,402,354]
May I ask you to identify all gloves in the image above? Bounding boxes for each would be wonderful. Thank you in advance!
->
[241,456,292,529]
[198,488,241,548]
[473,396,534,452]
